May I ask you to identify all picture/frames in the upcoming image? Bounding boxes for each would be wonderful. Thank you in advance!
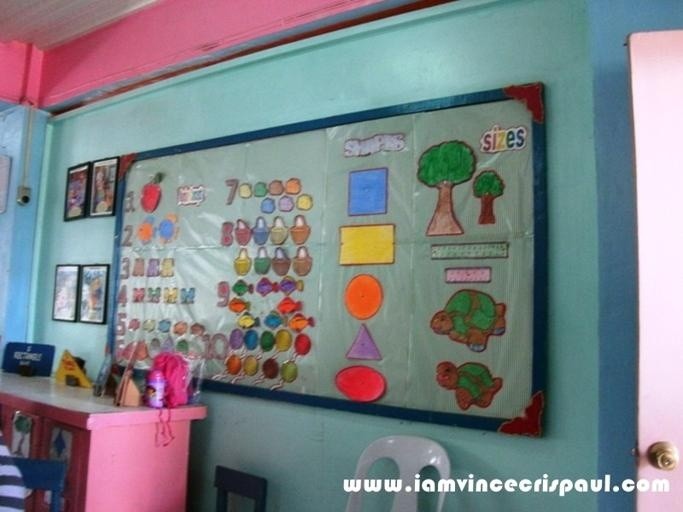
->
[50,263,79,323]
[88,155,120,218]
[62,161,93,223]
[76,263,110,325]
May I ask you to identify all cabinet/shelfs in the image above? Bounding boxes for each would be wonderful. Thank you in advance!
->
[0,368,209,512]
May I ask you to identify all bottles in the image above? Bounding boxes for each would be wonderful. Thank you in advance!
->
[147,370,166,410]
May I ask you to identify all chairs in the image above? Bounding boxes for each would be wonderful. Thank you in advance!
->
[213,465,267,512]
[344,430,453,512]
[10,455,66,511]
